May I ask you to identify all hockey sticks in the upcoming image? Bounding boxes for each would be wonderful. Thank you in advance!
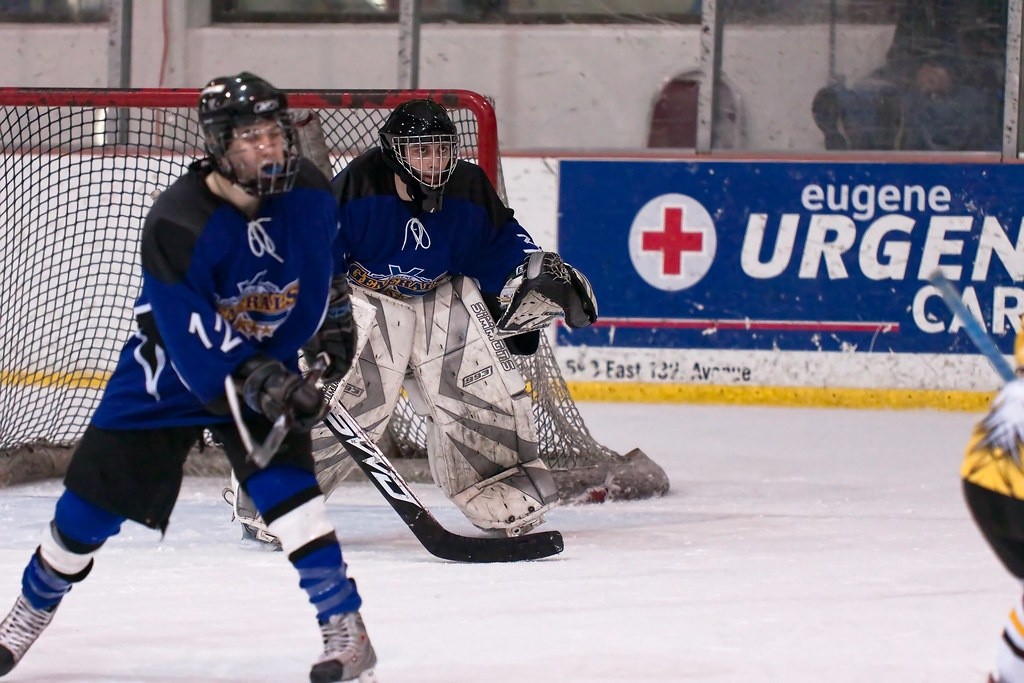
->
[224,351,328,468]
[150,186,565,563]
[929,269,1014,382]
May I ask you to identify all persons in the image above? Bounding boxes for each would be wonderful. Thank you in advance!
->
[962,321,1024,682]
[842,0,1009,153]
[233,98,599,545]
[0,68,380,683]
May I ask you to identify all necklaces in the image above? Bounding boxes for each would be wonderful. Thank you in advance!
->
[213,169,232,203]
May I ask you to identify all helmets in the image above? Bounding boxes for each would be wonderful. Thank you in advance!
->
[199,71,286,157]
[378,99,456,147]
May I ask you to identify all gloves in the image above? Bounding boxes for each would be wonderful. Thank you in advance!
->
[303,295,357,382]
[233,355,332,433]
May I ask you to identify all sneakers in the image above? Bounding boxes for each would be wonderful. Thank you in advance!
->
[0,594,58,678]
[310,610,377,682]
